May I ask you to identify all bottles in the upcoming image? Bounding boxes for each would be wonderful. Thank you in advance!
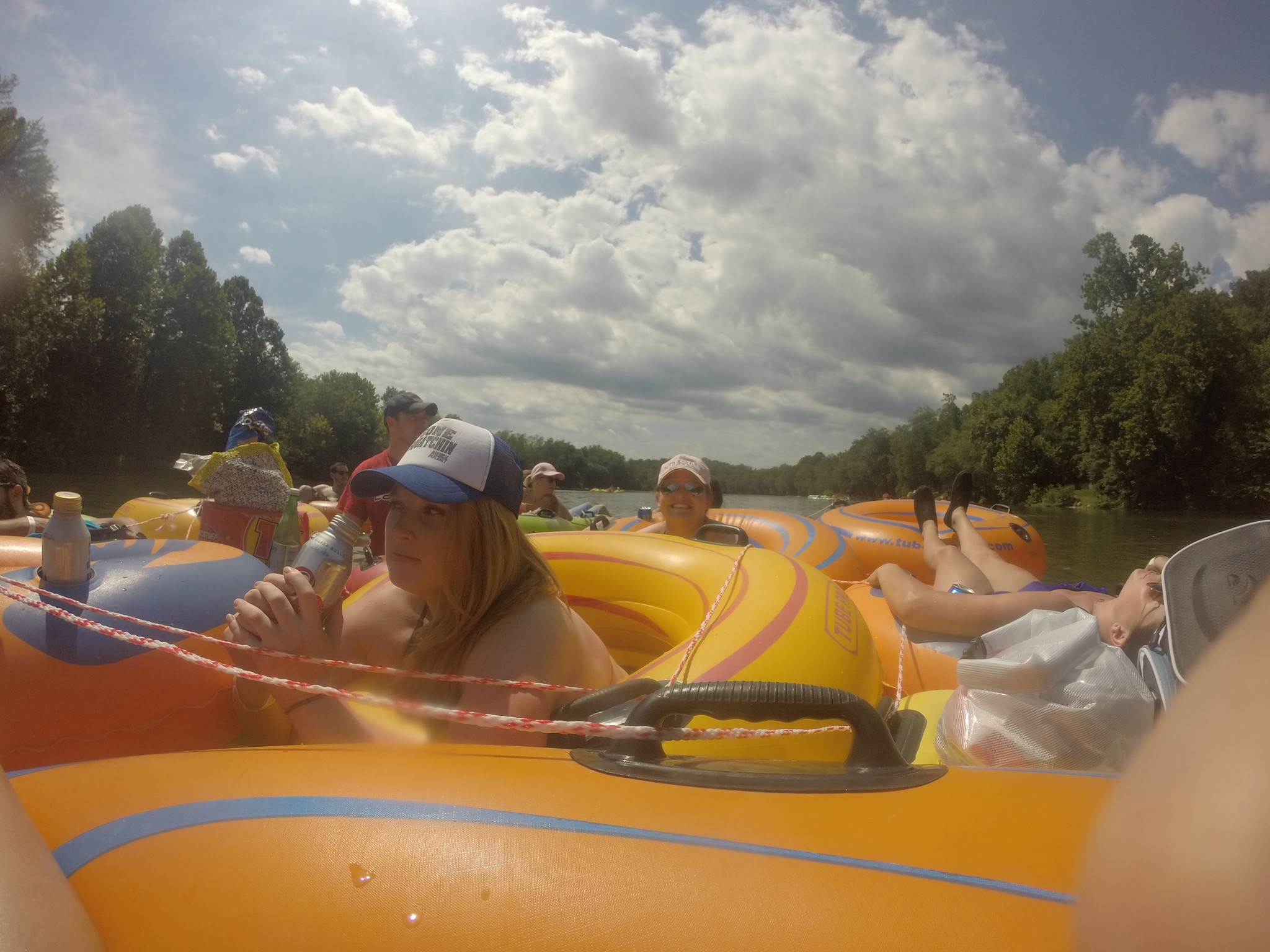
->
[269,487,300,573]
[288,512,362,629]
[41,490,91,584]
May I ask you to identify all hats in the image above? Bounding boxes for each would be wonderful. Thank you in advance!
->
[531,462,565,482]
[349,416,524,518]
[658,453,712,488]
[383,392,438,417]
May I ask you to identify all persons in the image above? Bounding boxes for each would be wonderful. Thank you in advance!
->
[863,470,1270,952]
[0,457,142,541]
[300,391,574,556]
[635,454,737,544]
[221,417,628,747]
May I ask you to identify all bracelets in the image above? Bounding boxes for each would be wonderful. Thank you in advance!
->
[1148,554,1168,572]
[282,691,327,714]
[26,516,36,535]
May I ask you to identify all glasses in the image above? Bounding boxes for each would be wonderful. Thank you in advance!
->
[659,479,704,497]
[335,470,350,476]
[1131,581,1163,639]
[541,477,560,483]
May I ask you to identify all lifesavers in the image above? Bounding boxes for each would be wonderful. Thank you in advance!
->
[0,498,1127,952]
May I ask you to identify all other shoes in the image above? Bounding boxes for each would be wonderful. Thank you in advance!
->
[941,470,974,532]
[913,485,939,537]
